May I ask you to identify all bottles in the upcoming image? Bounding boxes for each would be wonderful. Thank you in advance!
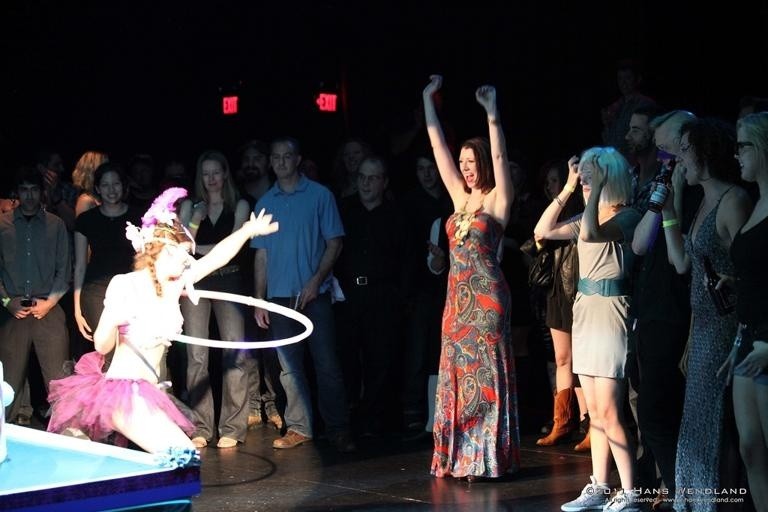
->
[701,254,734,316]
[647,156,677,212]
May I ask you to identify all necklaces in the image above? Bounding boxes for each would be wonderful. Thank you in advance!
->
[455,193,485,248]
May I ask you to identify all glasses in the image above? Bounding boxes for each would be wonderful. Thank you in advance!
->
[357,173,385,182]
[733,140,752,154]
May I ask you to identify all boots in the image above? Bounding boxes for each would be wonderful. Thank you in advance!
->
[573,415,592,452]
[535,388,575,448]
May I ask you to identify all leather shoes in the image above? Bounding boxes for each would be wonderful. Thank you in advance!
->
[465,470,483,485]
[243,415,264,428]
[272,427,312,450]
[270,416,283,428]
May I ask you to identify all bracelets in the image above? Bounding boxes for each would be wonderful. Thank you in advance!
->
[487,118,502,125]
[733,336,742,347]
[563,184,576,193]
[661,217,678,228]
[189,222,200,229]
[553,197,565,207]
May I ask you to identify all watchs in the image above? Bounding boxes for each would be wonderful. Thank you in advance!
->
[1,297,11,307]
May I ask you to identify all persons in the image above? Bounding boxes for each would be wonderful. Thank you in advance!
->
[700,112,768,512]
[330,138,373,200]
[238,140,277,210]
[72,150,112,263]
[647,115,754,512]
[45,186,279,457]
[506,146,535,436]
[339,154,405,422]
[424,75,520,484]
[127,157,160,208]
[179,149,250,449]
[600,64,658,147]
[0,190,20,217]
[534,148,641,512]
[535,153,593,452]
[622,110,651,179]
[250,130,359,455]
[0,164,72,427]
[72,162,142,368]
[630,105,701,512]
[403,148,450,435]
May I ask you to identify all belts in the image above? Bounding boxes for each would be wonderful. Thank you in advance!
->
[343,273,380,288]
[18,297,39,312]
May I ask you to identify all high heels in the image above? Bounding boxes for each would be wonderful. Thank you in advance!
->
[188,436,208,450]
[216,438,240,447]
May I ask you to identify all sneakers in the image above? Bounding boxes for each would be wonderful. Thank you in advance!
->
[557,475,610,512]
[603,490,641,512]
